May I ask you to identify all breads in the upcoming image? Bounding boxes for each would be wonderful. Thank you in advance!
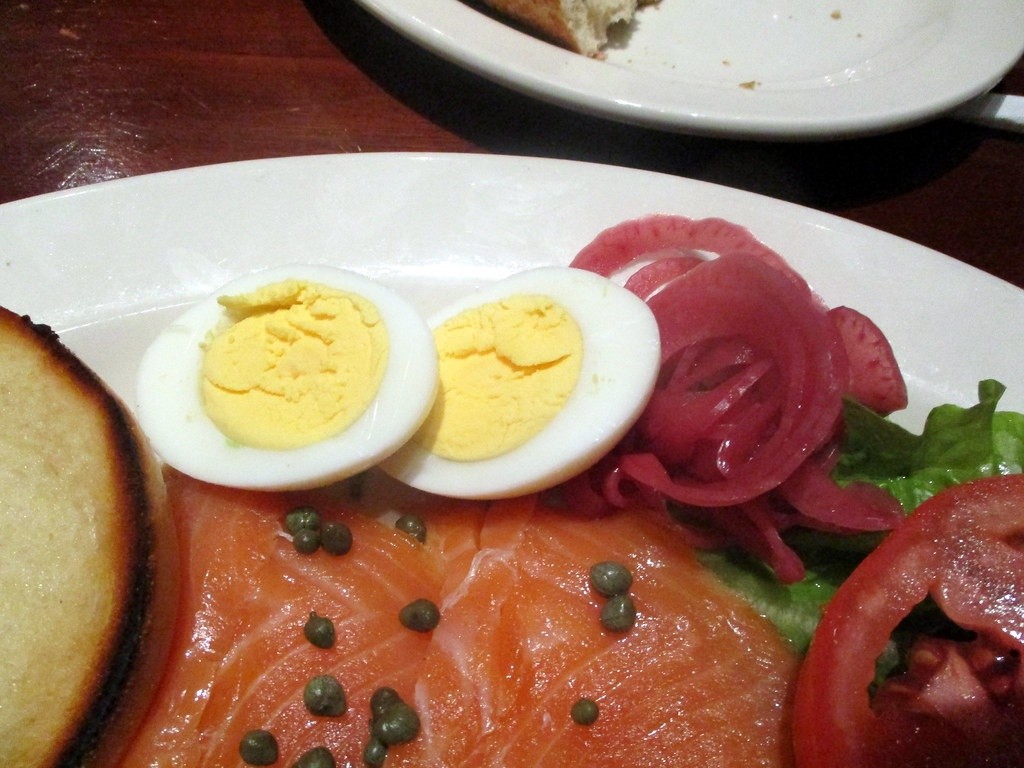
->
[484,0,655,59]
[0,308,179,768]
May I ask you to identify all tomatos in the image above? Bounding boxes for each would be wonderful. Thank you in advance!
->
[792,471,1024,768]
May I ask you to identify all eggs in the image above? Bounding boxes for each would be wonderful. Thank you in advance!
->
[374,265,664,500]
[134,263,439,493]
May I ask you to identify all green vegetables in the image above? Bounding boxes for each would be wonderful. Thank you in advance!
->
[689,372,1024,709]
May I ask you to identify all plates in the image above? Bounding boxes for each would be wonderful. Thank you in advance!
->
[0,154,1024,768]
[356,0,1024,143]
